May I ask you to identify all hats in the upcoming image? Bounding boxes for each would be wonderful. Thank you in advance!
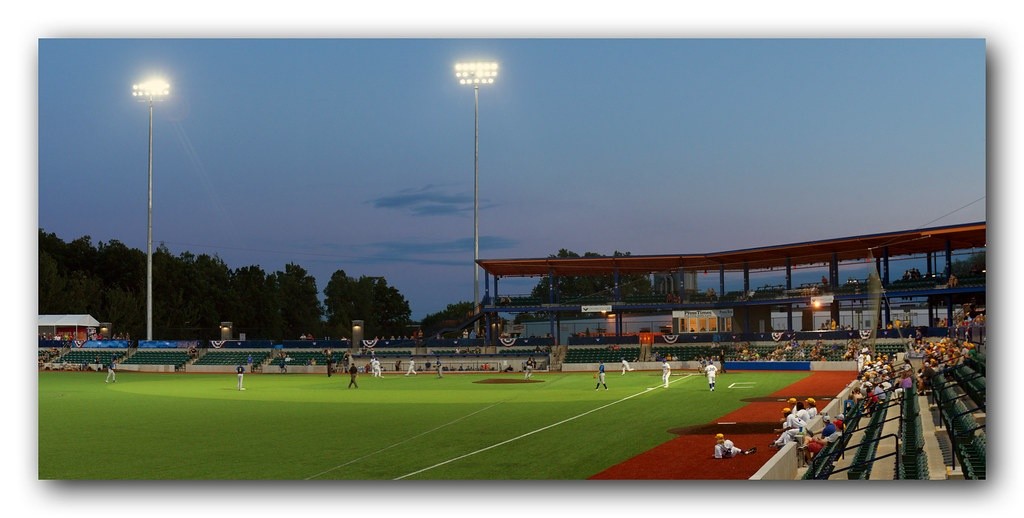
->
[714,433,724,439]
[780,408,791,413]
[786,397,797,403]
[709,361,713,364]
[662,358,666,362]
[836,414,844,420]
[861,352,891,381]
[805,398,816,404]
[822,415,830,422]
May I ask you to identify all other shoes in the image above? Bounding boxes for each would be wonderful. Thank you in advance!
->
[112,381,117,383]
[239,388,245,391]
[605,388,608,390]
[105,381,108,383]
[595,388,599,390]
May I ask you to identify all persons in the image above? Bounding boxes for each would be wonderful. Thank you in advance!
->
[236,364,245,390]
[277,328,490,378]
[695,351,727,377]
[593,359,609,390]
[949,273,959,290]
[733,311,986,467]
[501,295,512,304]
[902,268,923,280]
[712,433,757,459]
[585,327,590,337]
[38,332,130,383]
[247,353,253,365]
[349,363,360,388]
[706,288,716,303]
[704,361,718,392]
[620,358,634,376]
[822,275,828,286]
[666,292,681,304]
[504,357,543,379]
[662,358,671,387]
[655,351,678,360]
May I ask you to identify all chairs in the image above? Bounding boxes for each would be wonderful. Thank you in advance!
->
[798,352,987,480]
